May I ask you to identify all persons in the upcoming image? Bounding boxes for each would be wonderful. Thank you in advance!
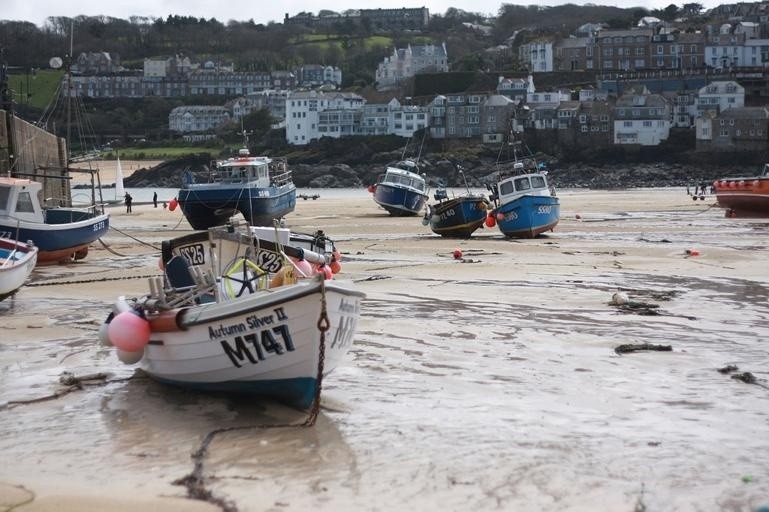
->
[153,192,157,208]
[290,247,313,277]
[685,177,715,195]
[124,192,133,213]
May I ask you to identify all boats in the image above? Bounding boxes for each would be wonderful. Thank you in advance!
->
[99,207,367,411]
[368,158,430,217]
[0,170,108,265]
[485,119,560,237]
[711,163,769,213]
[1,230,41,301]
[421,164,493,238]
[170,131,297,232]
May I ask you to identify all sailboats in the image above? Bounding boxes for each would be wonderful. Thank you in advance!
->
[95,157,126,204]
